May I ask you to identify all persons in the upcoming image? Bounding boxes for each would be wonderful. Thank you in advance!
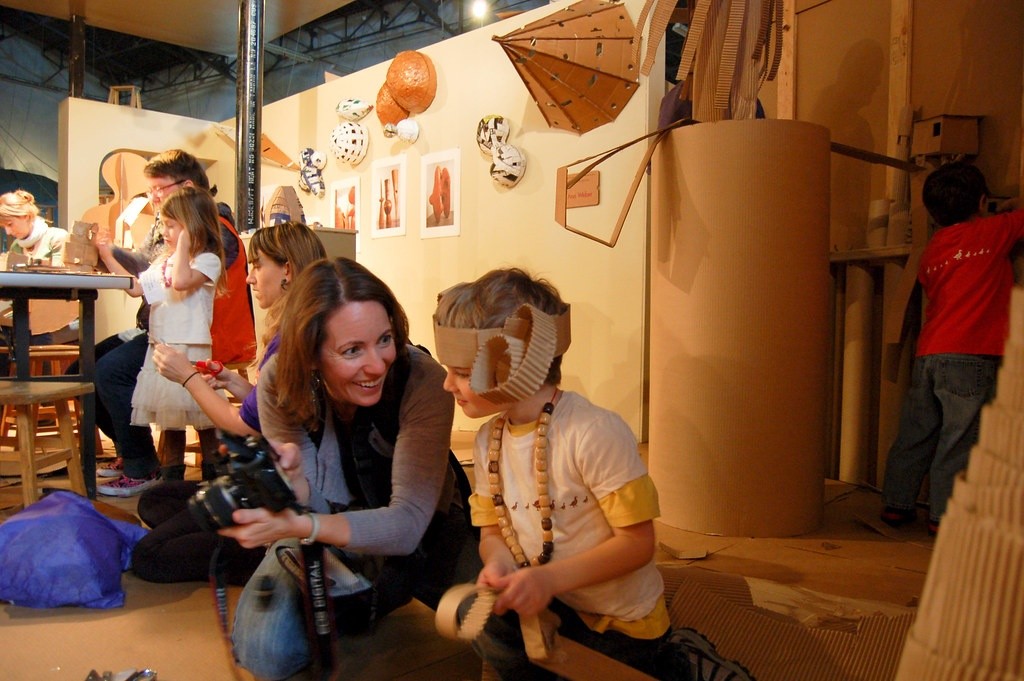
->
[63,148,258,496]
[217,256,474,681]
[431,264,758,681]
[97,186,229,488]
[1,188,71,428]
[879,160,1024,537]
[128,223,328,584]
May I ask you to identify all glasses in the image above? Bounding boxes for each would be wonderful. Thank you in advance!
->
[146,179,186,198]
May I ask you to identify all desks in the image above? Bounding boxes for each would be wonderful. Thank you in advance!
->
[0,270,135,501]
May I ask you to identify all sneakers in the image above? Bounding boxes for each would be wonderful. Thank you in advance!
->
[97,465,164,495]
[668,627,754,681]
[96,457,124,477]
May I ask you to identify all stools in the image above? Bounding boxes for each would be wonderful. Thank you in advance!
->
[158,362,249,469]
[107,86,143,109]
[0,381,97,511]
[0,345,105,455]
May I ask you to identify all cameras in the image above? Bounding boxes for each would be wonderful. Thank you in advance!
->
[187,434,297,534]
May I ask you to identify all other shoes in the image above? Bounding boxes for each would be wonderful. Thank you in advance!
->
[927,520,938,536]
[881,505,917,527]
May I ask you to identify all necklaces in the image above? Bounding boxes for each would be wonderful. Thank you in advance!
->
[487,388,558,569]
[159,251,173,288]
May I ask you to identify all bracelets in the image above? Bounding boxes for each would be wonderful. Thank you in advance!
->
[180,371,197,387]
[299,512,320,546]
